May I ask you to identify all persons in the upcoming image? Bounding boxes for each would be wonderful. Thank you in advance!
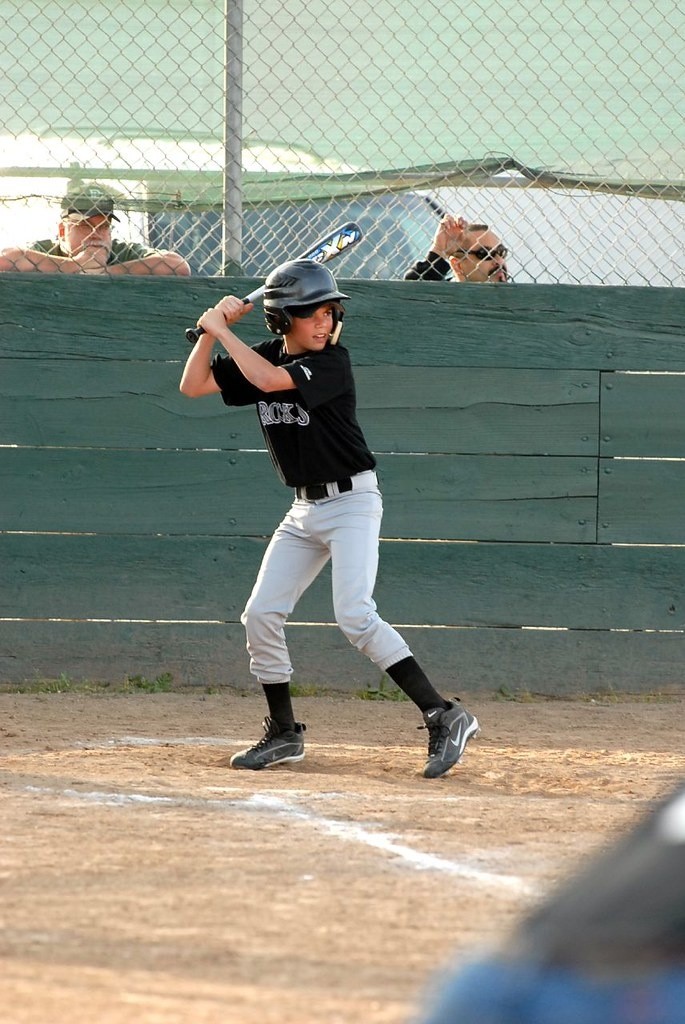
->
[404,215,509,282]
[179,259,481,779]
[0,184,191,276]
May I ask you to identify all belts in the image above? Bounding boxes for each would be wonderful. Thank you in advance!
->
[297,471,380,499]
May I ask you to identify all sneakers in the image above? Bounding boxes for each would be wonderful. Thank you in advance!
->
[230,716,307,770]
[417,697,478,778]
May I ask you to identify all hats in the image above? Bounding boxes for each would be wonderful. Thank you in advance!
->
[62,185,121,222]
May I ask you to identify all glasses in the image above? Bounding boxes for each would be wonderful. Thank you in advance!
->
[71,221,110,236]
[457,245,508,260]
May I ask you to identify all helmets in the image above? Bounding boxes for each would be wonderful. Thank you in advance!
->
[263,259,351,335]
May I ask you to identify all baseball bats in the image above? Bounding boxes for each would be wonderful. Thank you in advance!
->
[185,221,365,344]
